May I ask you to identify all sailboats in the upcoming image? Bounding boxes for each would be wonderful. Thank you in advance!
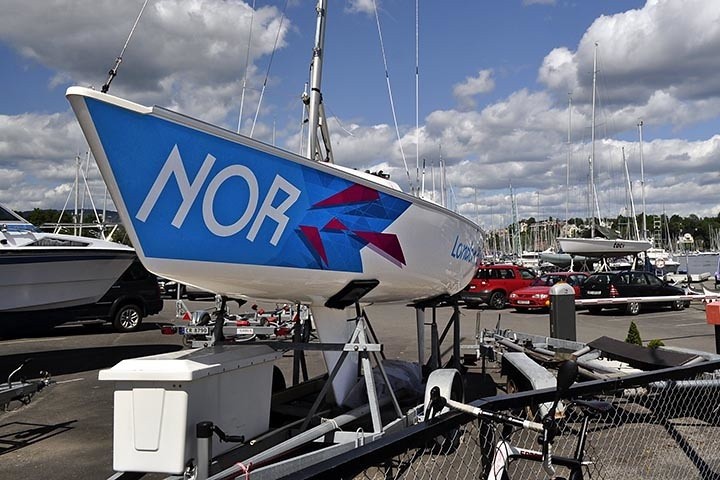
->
[59,1,490,311]
[457,40,711,316]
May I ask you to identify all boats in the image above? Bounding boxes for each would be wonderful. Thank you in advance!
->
[0,199,135,314]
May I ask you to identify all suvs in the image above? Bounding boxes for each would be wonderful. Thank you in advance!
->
[461,264,552,310]
[580,268,686,317]
[35,252,165,332]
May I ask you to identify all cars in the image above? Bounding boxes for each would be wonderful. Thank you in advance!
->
[507,271,592,314]
[155,275,230,301]
